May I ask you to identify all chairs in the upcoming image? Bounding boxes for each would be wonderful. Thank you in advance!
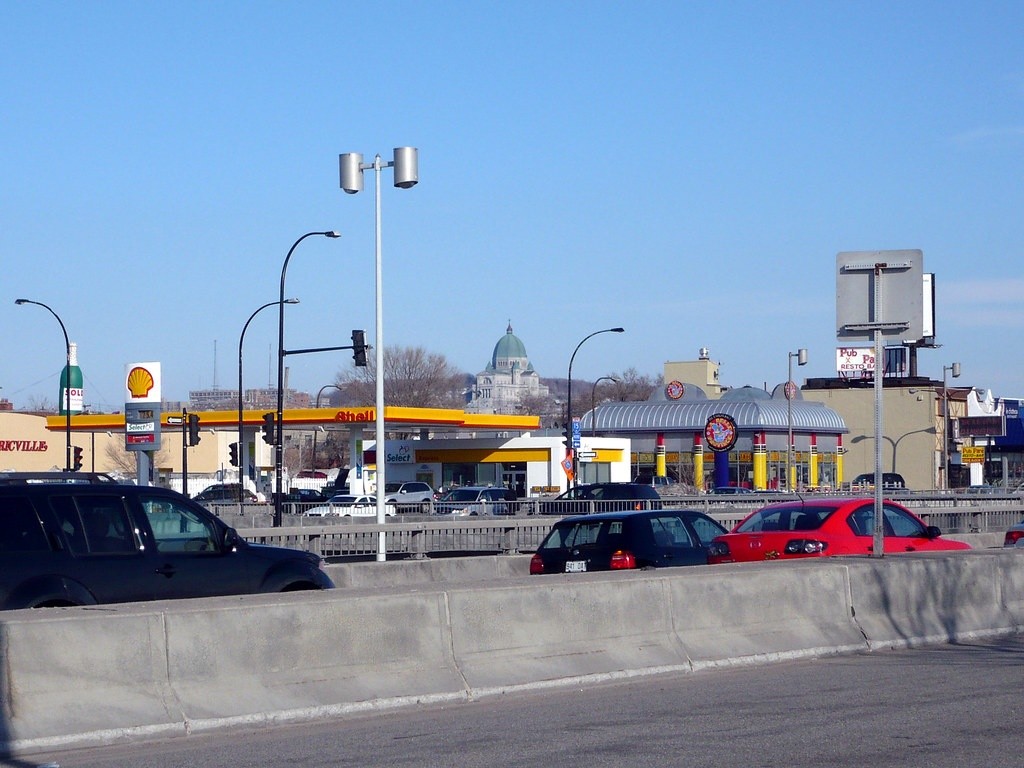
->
[655,530,676,547]
[793,515,817,530]
[604,532,622,545]
[56,506,127,549]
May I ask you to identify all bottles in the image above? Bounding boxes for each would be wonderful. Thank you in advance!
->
[58,343,83,416]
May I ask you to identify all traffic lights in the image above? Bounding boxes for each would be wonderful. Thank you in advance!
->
[74,444,83,472]
[228,443,238,465]
[262,411,275,444]
[562,421,572,447]
[189,414,201,445]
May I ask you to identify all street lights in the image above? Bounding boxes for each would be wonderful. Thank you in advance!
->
[275,231,343,529]
[13,295,72,471]
[567,328,626,498]
[237,297,300,516]
[908,388,988,492]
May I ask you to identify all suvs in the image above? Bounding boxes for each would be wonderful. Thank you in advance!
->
[0,481,337,615]
[0,470,120,484]
[528,508,729,577]
[846,471,906,490]
[705,487,755,504]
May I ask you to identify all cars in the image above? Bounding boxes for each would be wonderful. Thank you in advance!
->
[706,497,974,565]
[753,490,796,503]
[187,477,664,517]
[1003,516,1024,548]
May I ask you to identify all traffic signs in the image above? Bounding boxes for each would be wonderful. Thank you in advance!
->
[166,416,183,425]
